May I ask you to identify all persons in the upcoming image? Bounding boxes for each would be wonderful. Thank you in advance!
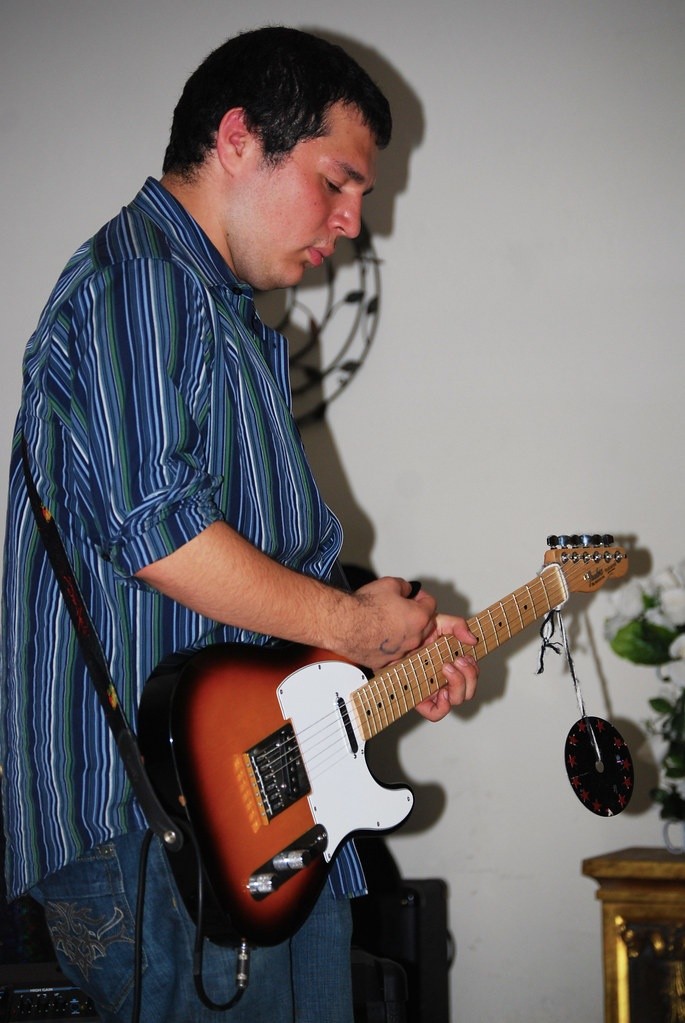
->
[0,29,481,1023]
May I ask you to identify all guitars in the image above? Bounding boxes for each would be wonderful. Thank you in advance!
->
[138,529,632,955]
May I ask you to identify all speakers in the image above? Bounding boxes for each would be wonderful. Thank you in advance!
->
[350,879,451,1023]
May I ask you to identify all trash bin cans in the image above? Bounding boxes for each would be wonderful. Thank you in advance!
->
[582,846,685,1023]
